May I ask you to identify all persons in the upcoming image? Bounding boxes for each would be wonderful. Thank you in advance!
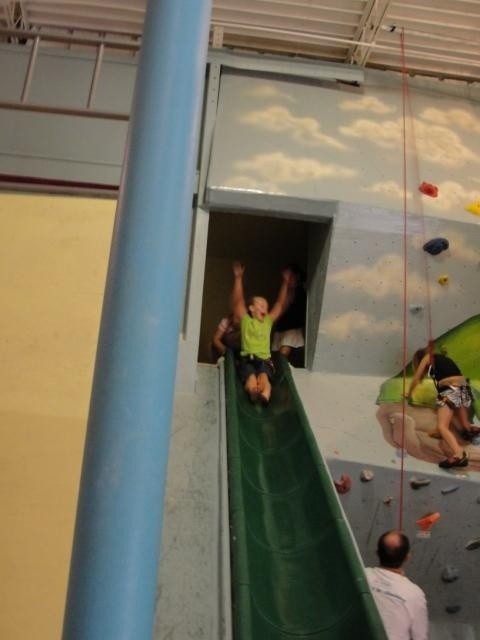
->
[401,347,480,469]
[210,312,241,365]
[228,260,299,409]
[362,529,429,640]
[271,273,306,357]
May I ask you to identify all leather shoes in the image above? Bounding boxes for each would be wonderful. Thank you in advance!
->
[462,431,474,440]
[439,456,468,468]
